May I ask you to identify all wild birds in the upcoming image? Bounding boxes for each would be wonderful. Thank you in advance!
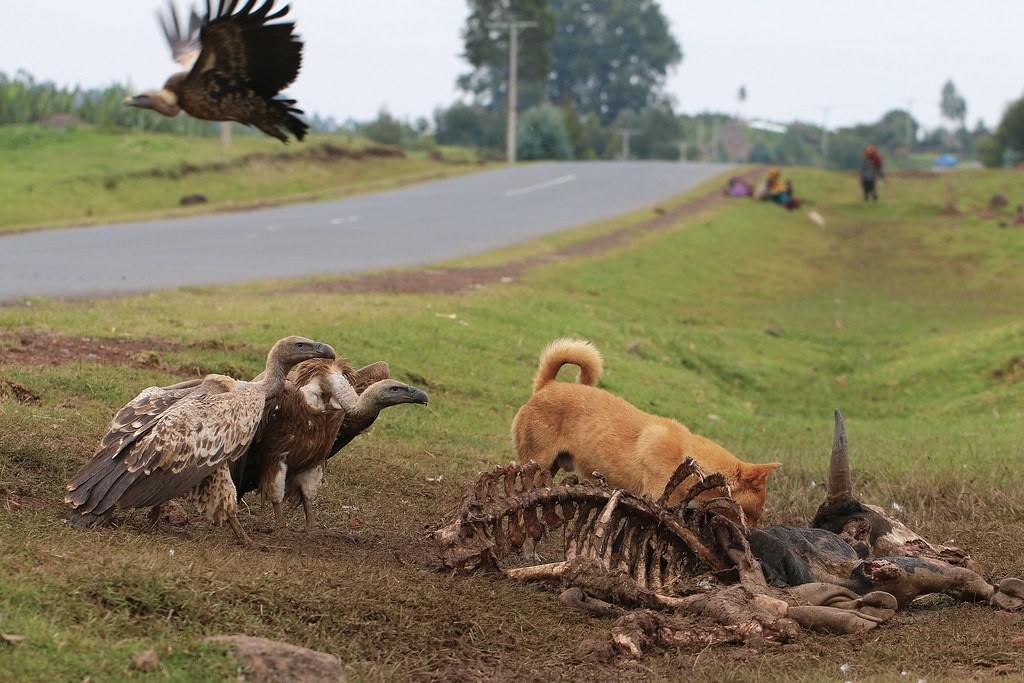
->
[125,0,312,146]
[63,333,339,542]
[238,355,431,528]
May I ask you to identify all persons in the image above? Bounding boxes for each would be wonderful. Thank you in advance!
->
[724,169,803,210]
[860,145,882,202]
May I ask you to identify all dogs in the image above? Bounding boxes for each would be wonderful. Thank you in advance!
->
[510,337,782,563]
